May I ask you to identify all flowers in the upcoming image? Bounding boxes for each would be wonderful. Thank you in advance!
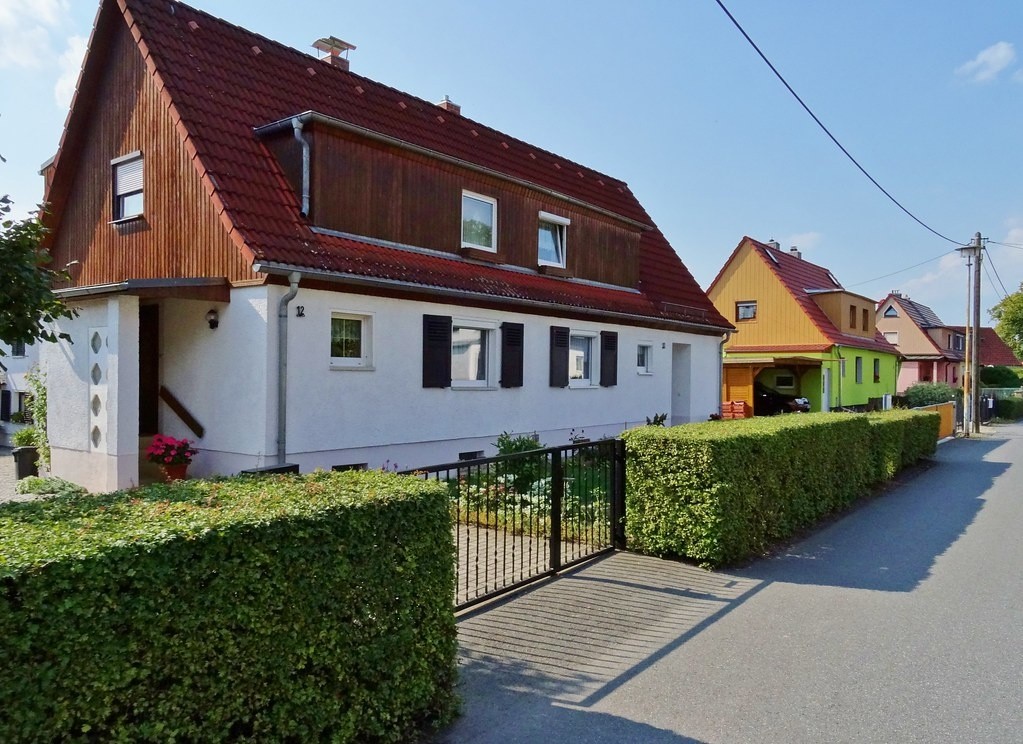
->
[144,433,199,466]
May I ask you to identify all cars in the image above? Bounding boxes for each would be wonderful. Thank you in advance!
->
[755,381,811,419]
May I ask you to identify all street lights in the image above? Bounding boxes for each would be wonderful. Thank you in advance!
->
[955,244,980,437]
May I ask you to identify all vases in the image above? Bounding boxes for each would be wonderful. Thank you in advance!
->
[152,463,188,484]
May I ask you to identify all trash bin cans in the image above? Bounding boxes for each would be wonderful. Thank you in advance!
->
[12,445,39,480]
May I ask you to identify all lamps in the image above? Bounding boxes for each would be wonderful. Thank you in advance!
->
[205,309,219,329]
